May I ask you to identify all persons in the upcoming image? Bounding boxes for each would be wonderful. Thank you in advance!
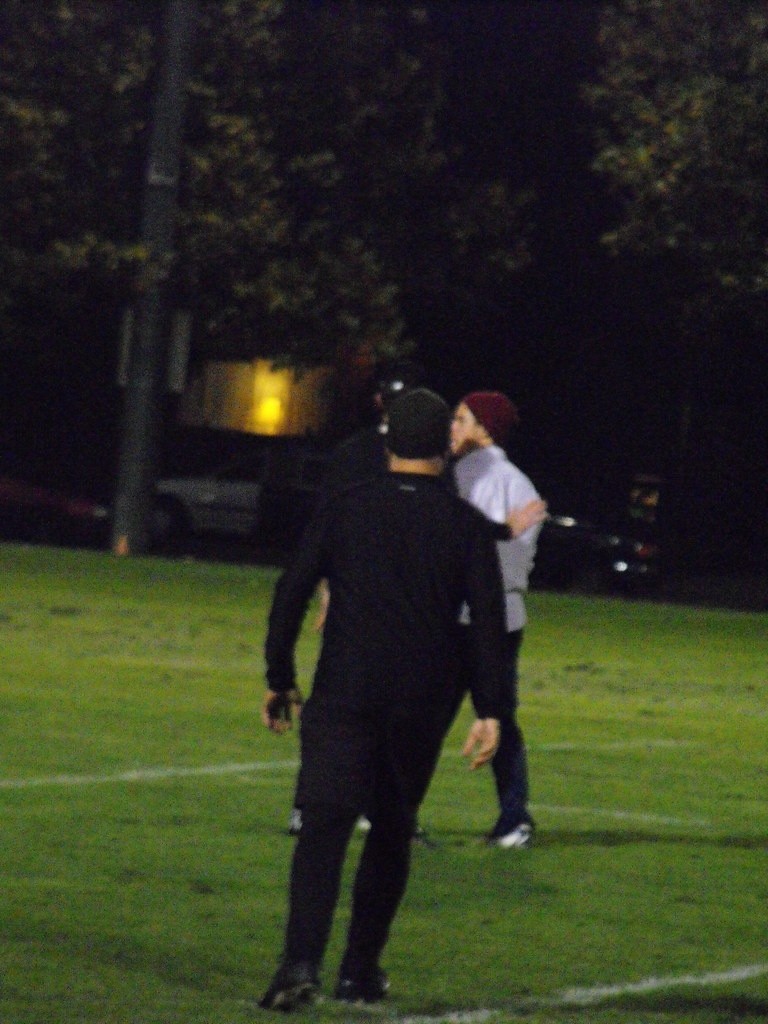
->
[359,393,543,847]
[261,392,504,1011]
[605,484,658,597]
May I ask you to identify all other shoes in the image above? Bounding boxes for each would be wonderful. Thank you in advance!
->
[336,968,391,1005]
[491,811,534,850]
[261,978,321,1013]
[286,808,303,836]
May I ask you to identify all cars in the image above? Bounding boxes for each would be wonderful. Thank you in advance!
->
[527,491,665,594]
[0,469,117,552]
[141,443,330,555]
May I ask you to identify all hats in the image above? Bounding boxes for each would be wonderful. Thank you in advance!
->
[388,389,449,455]
[463,392,520,444]
